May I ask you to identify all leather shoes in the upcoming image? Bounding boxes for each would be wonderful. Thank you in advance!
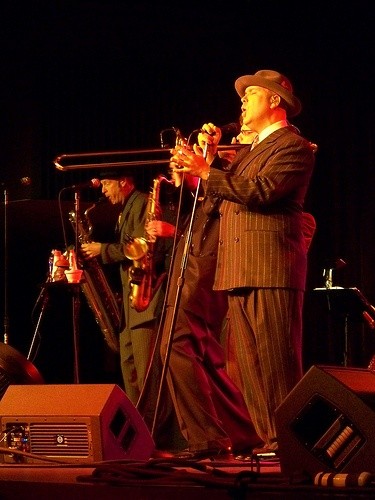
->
[234,449,281,461]
[165,443,234,461]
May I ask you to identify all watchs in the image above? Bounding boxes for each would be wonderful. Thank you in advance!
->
[201,165,210,183]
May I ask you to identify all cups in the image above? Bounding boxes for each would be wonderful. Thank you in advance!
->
[322,269,333,288]
[64,269,82,283]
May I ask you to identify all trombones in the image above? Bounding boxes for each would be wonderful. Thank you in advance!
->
[53,140,318,171]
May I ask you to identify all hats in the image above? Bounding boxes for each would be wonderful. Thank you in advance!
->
[234,70,301,120]
[95,161,133,179]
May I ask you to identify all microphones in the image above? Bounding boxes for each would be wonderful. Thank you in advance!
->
[0,178,33,188]
[63,179,101,191]
[196,123,241,136]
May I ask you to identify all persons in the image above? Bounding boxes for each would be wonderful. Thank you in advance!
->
[80,165,188,455]
[147,133,264,464]
[168,70,317,466]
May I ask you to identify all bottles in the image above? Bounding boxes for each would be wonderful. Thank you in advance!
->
[69,244,78,271]
[51,251,64,282]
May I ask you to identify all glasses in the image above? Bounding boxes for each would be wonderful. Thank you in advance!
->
[239,128,254,135]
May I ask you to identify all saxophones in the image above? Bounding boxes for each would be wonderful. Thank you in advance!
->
[55,196,122,355]
[122,173,176,314]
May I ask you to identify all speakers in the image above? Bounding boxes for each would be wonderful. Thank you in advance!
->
[273,364,375,484]
[0,383,157,468]
